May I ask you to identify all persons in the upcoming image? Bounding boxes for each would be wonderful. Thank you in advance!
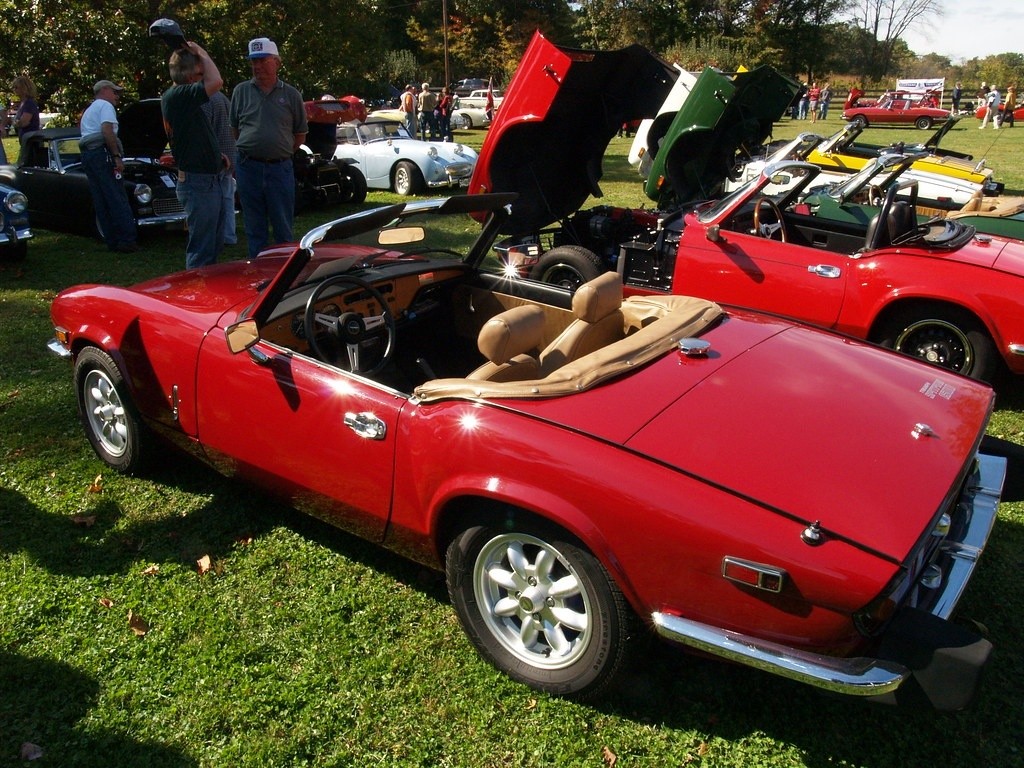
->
[13,76,44,146]
[951,82,963,114]
[229,38,308,260]
[400,83,460,142]
[161,40,231,271]
[997,87,1016,127]
[201,91,237,246]
[792,83,833,123]
[617,121,631,139]
[0,101,9,165]
[978,85,1000,130]
[79,79,138,253]
[977,82,986,109]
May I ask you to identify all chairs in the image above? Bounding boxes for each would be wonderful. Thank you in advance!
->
[904,100,912,109]
[465,305,546,382]
[857,180,919,254]
[538,271,625,377]
[970,190,984,207]
[962,197,983,212]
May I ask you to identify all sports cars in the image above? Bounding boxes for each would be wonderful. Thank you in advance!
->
[434,75,507,130]
[47,188,1007,700]
[332,116,482,196]
[628,63,1024,244]
[1,99,190,241]
[464,26,1024,388]
[158,95,369,215]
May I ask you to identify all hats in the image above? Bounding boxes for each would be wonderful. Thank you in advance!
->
[94,80,124,95]
[148,18,191,49]
[247,38,281,59]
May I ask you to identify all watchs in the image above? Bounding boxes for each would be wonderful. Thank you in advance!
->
[112,153,122,158]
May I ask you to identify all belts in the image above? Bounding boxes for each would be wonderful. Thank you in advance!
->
[251,158,287,167]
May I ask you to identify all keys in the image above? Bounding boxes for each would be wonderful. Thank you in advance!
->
[104,148,112,163]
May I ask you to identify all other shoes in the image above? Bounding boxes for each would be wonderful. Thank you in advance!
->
[117,242,142,253]
